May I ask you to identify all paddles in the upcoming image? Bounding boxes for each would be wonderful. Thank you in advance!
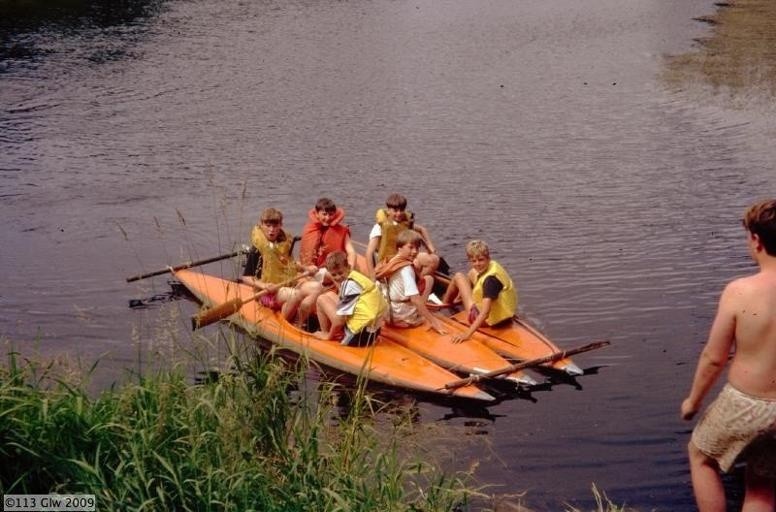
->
[445,339,610,390]
[191,270,309,333]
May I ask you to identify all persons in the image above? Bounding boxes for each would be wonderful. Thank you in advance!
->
[366,194,439,282]
[376,229,449,334]
[299,198,357,292]
[443,240,518,343]
[313,250,388,347]
[680,197,776,512]
[243,208,322,330]
